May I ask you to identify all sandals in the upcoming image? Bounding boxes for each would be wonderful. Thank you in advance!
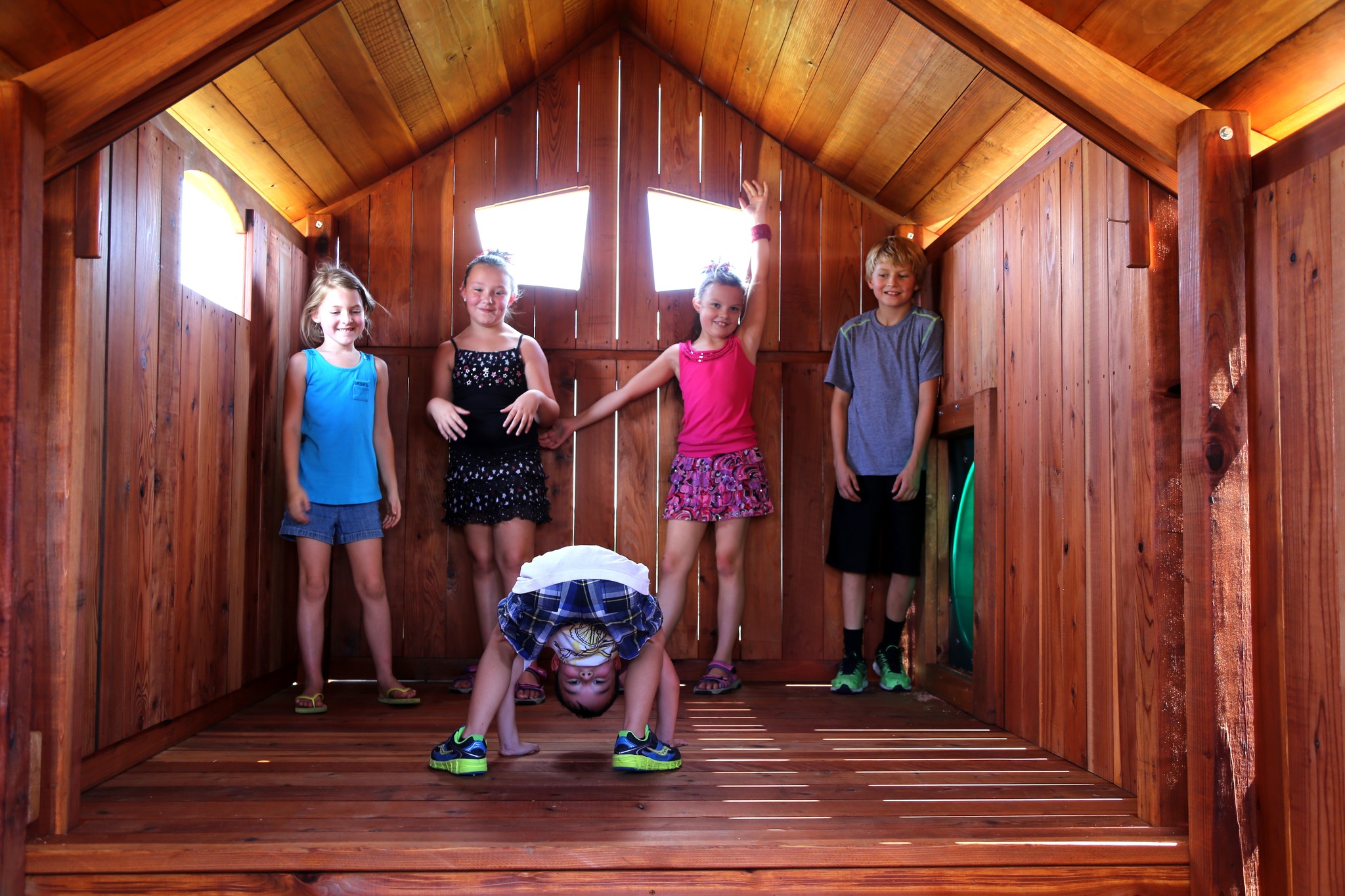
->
[448,667,479,694]
[693,660,743,696]
[513,664,547,705]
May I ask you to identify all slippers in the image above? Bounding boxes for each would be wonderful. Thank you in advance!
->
[378,685,420,704]
[295,693,327,714]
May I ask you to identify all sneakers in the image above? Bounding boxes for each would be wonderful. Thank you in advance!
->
[871,643,911,692]
[429,725,488,775]
[612,725,682,772]
[829,648,868,693]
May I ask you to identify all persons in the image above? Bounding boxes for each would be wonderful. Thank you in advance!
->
[429,545,682,775]
[822,235,943,694]
[539,180,774,694]
[425,249,560,702]
[278,256,422,713]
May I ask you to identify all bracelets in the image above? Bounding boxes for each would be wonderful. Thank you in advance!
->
[751,223,772,241]
